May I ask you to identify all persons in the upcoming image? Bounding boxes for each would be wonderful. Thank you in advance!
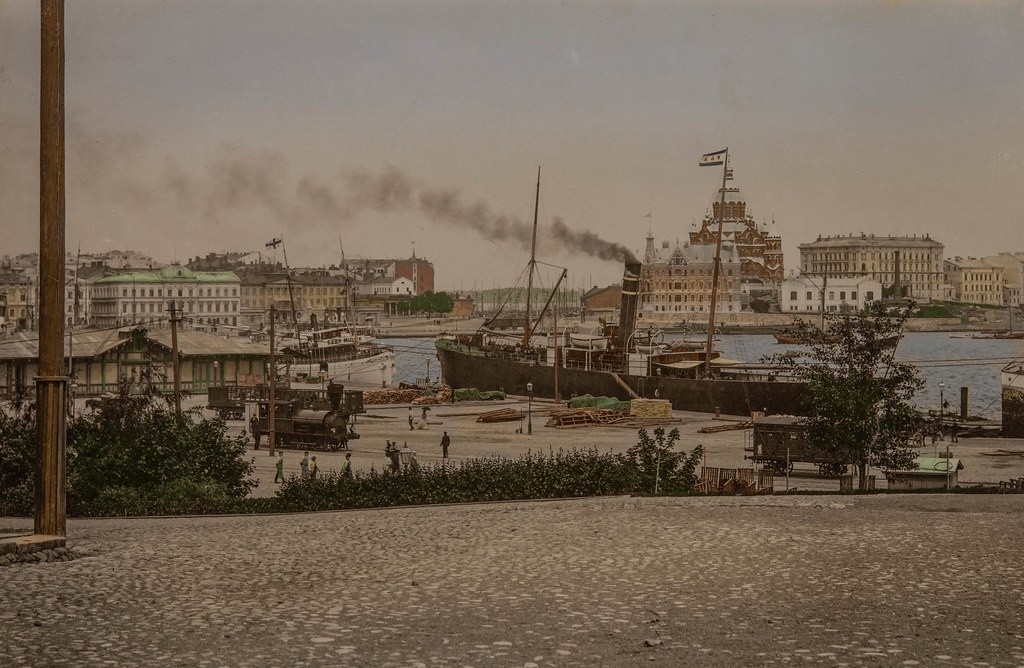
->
[929,419,958,445]
[343,454,353,478]
[408,407,414,431]
[300,451,309,480]
[309,456,322,480]
[274,451,287,483]
[449,333,542,364]
[942,399,950,415]
[416,409,428,430]
[383,438,400,473]
[250,413,261,450]
[439,432,450,458]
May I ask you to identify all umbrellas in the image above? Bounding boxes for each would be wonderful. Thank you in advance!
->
[422,406,431,411]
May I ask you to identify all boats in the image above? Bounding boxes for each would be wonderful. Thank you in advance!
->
[219,232,397,388]
[433,145,906,418]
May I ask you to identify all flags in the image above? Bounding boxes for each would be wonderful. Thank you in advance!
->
[699,149,727,167]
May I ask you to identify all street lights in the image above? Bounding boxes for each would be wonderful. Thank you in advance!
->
[939,381,945,421]
[426,357,431,380]
[214,361,218,385]
[526,383,533,434]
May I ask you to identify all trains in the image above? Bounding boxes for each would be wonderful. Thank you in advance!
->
[255,382,361,453]
